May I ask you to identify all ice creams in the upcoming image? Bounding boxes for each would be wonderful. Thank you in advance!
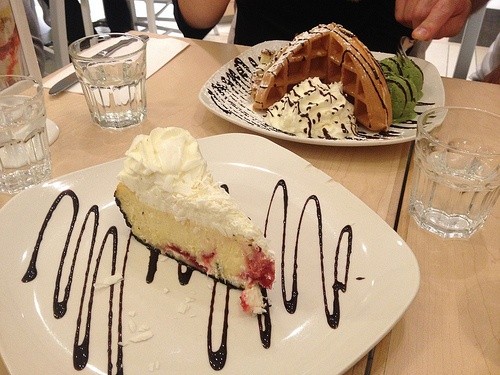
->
[377,52,423,123]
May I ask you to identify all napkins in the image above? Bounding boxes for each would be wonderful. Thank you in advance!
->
[42,36,191,107]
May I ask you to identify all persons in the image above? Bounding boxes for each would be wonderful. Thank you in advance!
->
[172,0,490,56]
[44,0,134,64]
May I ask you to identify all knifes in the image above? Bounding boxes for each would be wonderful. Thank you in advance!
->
[49,34,149,95]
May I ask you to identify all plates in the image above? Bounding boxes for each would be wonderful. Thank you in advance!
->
[0,132,420,375]
[199,40,448,146]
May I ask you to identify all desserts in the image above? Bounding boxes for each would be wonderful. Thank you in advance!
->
[266,76,359,139]
[112,124,275,316]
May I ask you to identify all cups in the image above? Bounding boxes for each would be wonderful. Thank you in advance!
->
[0,75,52,195]
[68,33,148,130]
[409,106,500,239]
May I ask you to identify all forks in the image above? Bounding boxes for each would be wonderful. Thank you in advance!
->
[396,36,418,60]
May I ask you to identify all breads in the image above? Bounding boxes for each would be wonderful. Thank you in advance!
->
[325,23,392,134]
[254,23,336,110]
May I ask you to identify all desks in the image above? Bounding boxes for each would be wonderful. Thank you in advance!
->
[0,30,500,375]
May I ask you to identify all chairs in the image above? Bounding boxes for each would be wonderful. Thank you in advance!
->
[9,0,491,85]
